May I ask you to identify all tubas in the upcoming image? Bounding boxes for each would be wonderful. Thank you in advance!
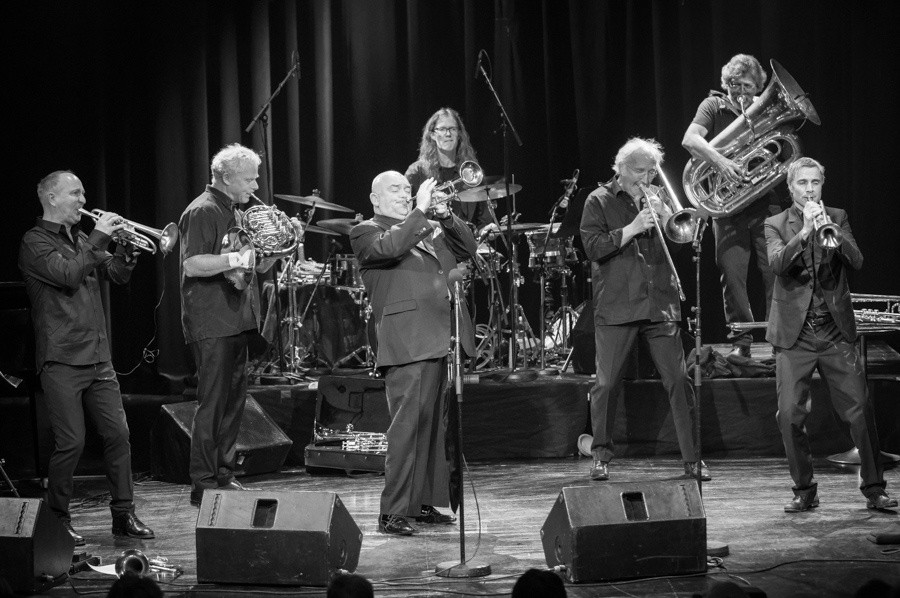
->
[681,56,823,220]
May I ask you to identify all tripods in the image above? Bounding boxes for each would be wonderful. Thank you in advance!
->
[266,242,378,379]
[468,225,573,377]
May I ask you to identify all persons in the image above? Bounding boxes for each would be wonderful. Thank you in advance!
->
[176,141,303,506]
[761,155,899,513]
[350,169,480,537]
[403,106,488,375]
[17,170,155,544]
[579,135,717,482]
[326,573,373,598]
[681,53,786,366]
[108,570,165,597]
[512,567,567,598]
[691,577,900,598]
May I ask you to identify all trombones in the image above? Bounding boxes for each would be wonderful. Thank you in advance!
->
[639,162,701,301]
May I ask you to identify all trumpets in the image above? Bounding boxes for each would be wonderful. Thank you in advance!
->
[806,195,845,250]
[402,160,484,210]
[78,206,179,257]
[312,416,389,454]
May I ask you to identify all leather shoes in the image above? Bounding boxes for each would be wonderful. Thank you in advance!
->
[784,494,819,512]
[378,514,420,536]
[222,480,265,491]
[189,498,202,506]
[416,504,457,524]
[866,493,899,508]
[112,513,155,539]
[590,458,610,480]
[725,344,751,358]
[63,519,87,546]
[684,460,712,481]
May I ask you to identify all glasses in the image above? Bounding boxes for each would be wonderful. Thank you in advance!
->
[626,167,658,182]
[433,126,459,135]
[728,80,756,92]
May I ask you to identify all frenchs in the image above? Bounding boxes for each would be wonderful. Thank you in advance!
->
[219,192,300,292]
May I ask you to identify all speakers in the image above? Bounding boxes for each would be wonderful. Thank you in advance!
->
[150,393,296,482]
[537,477,709,585]
[569,300,661,380]
[1,497,79,588]
[193,487,362,588]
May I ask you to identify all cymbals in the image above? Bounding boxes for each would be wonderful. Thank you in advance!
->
[486,221,546,233]
[453,182,523,202]
[315,213,364,235]
[274,188,355,213]
[302,223,343,237]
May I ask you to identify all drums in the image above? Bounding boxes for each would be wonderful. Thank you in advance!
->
[523,222,580,269]
[327,250,367,293]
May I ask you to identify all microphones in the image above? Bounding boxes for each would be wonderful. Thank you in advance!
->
[448,267,461,302]
[691,207,708,248]
[292,48,301,80]
[560,173,578,209]
[329,237,346,251]
[474,51,484,81]
